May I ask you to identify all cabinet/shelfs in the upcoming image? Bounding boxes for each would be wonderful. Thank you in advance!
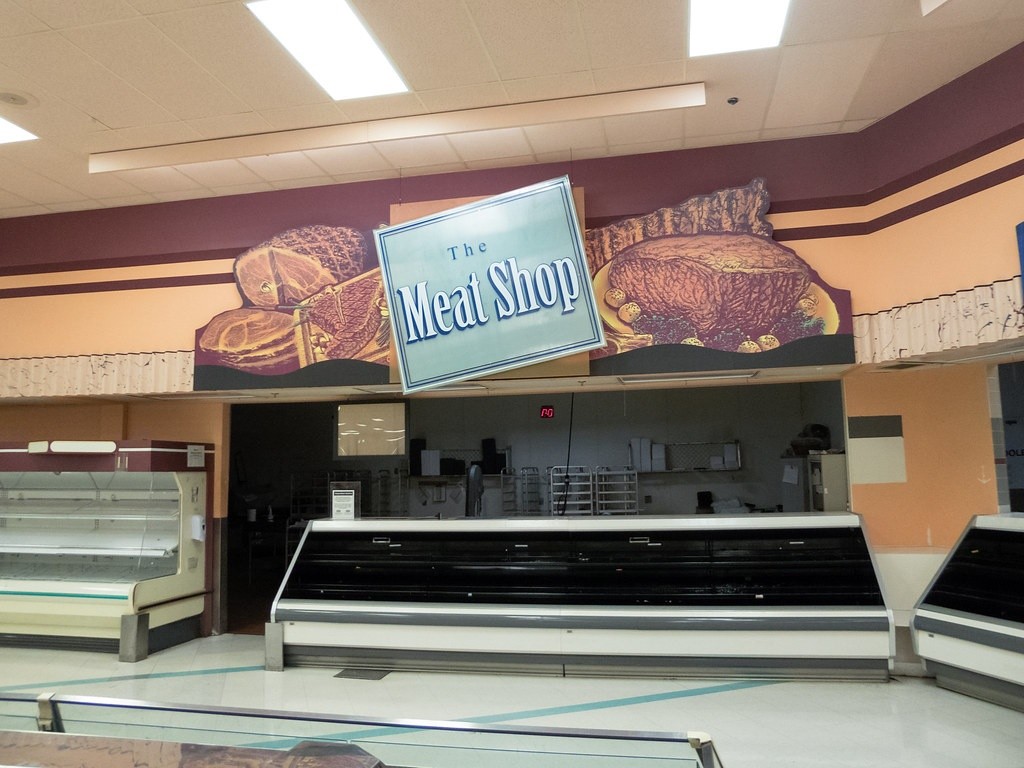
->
[0,440,214,663]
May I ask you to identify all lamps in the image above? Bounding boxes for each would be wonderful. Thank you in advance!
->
[86,79,711,175]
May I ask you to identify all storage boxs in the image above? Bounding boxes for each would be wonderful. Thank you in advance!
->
[631,437,666,472]
[470,438,507,475]
[807,454,847,512]
[409,438,465,476]
[710,444,738,469]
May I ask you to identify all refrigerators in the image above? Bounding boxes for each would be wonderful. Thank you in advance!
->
[807,453,850,512]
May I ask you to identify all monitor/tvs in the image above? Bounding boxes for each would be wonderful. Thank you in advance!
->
[333,397,410,468]
